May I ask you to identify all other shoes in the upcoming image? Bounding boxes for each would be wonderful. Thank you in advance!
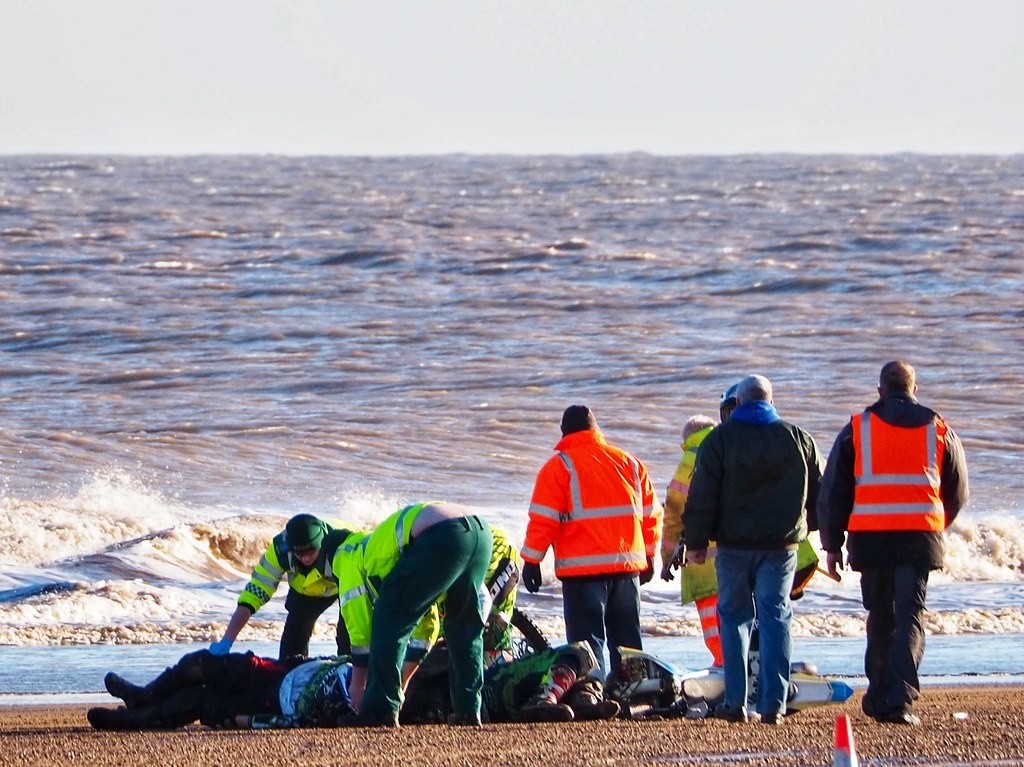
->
[335,706,402,730]
[450,714,485,730]
[713,701,749,724]
[759,712,787,725]
[86,705,137,732]
[102,672,145,706]
[893,710,924,726]
[519,691,576,722]
[859,691,886,723]
[570,691,620,721]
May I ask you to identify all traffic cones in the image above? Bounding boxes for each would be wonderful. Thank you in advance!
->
[832,712,859,767]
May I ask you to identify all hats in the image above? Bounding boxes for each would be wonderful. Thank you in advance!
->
[719,383,742,412]
[557,403,599,432]
[288,515,333,554]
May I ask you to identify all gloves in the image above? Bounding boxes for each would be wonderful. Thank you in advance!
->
[640,555,664,589]
[660,558,679,583]
[209,637,233,656]
[521,561,543,594]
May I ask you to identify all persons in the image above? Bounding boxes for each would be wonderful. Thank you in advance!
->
[520,405,662,689]
[436,526,520,648]
[86,649,354,732]
[322,501,493,729]
[659,374,825,723]
[209,513,356,661]
[481,625,620,723]
[820,360,968,722]
